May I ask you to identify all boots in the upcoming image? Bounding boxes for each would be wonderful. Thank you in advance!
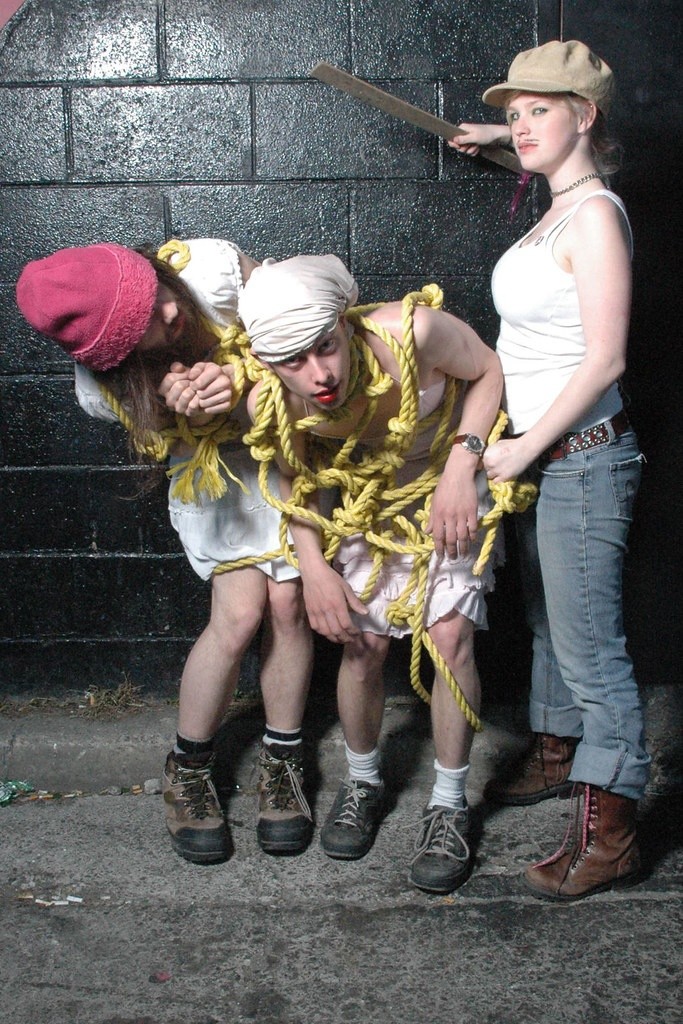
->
[520,781,641,902]
[484,732,581,805]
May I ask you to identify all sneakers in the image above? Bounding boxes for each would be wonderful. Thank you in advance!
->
[162,750,233,863]
[257,739,315,851]
[408,795,471,892]
[321,772,386,858]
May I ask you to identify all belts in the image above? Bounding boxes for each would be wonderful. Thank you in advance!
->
[539,409,630,460]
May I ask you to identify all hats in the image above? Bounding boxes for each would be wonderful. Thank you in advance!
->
[482,40,615,122]
[17,243,158,372]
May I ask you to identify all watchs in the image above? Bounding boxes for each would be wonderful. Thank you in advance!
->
[450,434,486,458]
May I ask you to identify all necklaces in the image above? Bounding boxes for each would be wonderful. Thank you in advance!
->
[548,172,610,198]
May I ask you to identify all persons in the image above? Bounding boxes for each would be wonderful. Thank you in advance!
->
[15,40,652,900]
